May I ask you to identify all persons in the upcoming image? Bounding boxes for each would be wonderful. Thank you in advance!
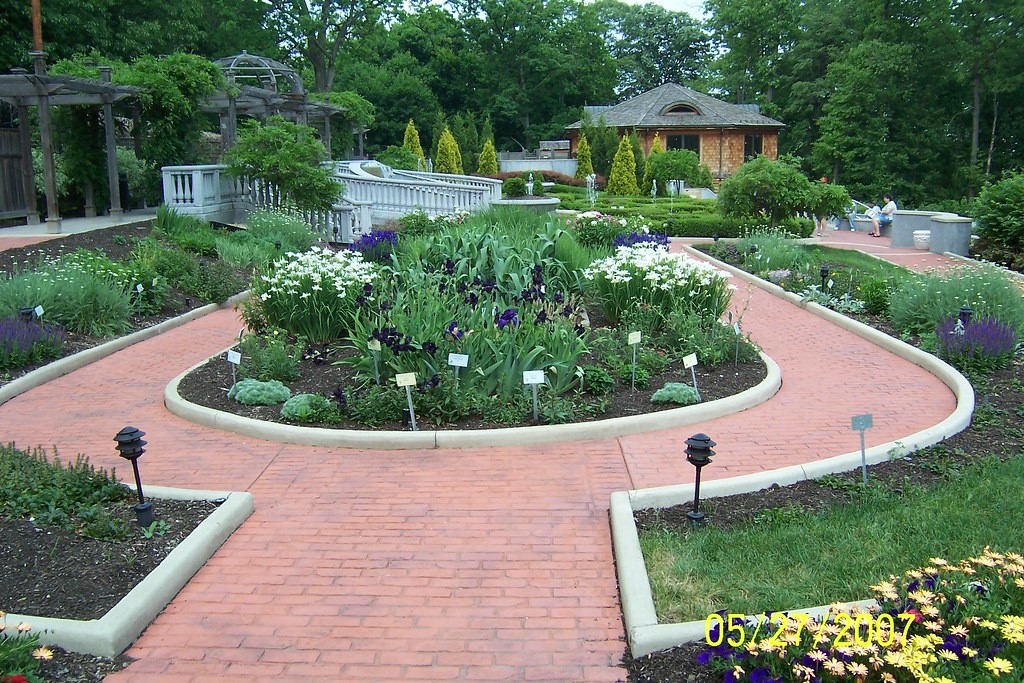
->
[817,210,831,237]
[799,212,815,238]
[833,202,857,231]
[864,201,880,219]
[868,194,897,237]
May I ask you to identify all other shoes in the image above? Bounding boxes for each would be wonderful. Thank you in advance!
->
[833,227,838,230]
[868,232,875,235]
[822,233,829,237]
[871,234,881,237]
[818,232,822,236]
[851,228,855,232]
